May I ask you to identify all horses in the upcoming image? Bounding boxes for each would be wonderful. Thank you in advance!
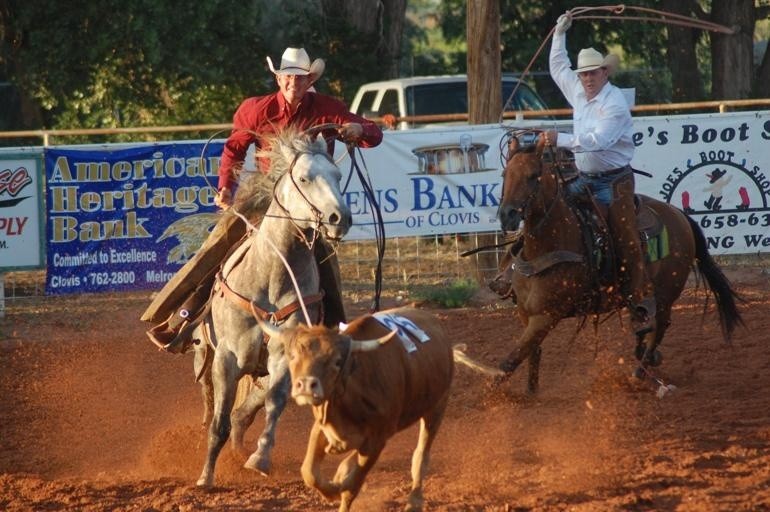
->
[487,132,750,397]
[190,121,355,490]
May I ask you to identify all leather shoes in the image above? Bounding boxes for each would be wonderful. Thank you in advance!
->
[486,278,516,305]
[152,328,176,343]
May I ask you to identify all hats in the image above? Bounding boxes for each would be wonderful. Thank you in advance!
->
[265,46,325,89]
[573,47,620,74]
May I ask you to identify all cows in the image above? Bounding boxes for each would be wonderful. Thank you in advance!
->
[249,299,505,512]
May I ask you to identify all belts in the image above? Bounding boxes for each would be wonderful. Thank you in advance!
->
[580,164,630,178]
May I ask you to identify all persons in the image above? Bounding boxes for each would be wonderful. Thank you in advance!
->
[146,44,383,354]
[487,10,657,335]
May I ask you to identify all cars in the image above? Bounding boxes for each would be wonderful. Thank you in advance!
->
[343,74,556,123]
